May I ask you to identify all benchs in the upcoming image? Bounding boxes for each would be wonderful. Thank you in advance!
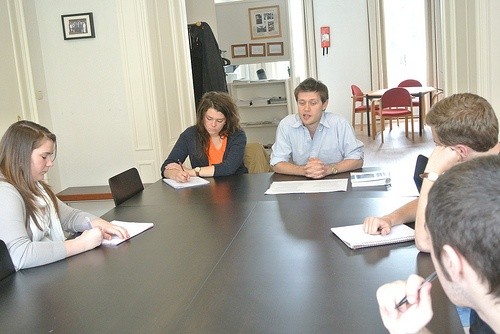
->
[56,186,113,201]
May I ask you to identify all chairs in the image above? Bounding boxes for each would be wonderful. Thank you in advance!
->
[108,167,144,207]
[351,79,422,143]
[243,143,269,173]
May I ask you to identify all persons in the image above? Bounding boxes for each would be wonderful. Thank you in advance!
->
[376,154,500,334]
[361,93,500,253]
[161,90,249,183]
[269,77,365,179]
[0,120,130,272]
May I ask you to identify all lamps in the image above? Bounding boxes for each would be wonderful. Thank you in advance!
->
[320,27,330,56]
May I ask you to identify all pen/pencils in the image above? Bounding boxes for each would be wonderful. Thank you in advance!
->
[396,272,437,309]
[177,158,184,171]
[86,216,94,228]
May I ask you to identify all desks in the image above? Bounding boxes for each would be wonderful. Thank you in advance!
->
[361,87,436,140]
[0,166,465,334]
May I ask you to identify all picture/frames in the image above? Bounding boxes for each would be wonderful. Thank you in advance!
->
[61,12,95,40]
[231,44,248,58]
[266,42,284,56]
[249,43,265,57]
[248,5,282,40]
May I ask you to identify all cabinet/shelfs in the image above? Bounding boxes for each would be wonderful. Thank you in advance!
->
[229,77,292,154]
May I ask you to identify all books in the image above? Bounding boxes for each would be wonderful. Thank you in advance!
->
[350,169,391,188]
[330,224,415,249]
[235,97,286,107]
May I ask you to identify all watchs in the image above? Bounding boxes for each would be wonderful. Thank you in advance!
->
[194,167,201,177]
[330,163,338,175]
[419,172,440,182]
